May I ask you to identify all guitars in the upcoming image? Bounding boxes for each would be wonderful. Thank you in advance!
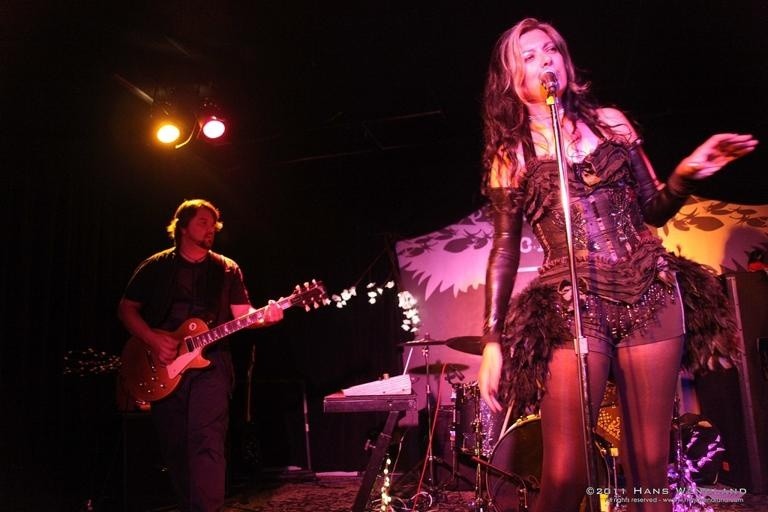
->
[119,279,332,402]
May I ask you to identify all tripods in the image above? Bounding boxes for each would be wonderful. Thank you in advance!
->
[372,345,491,506]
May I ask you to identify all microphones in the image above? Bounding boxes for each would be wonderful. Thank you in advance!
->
[519,482,528,512]
[540,68,557,93]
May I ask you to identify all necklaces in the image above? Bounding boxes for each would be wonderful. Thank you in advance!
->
[525,108,566,121]
[179,248,208,264]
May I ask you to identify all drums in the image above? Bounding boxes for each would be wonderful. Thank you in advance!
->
[418,405,454,453]
[458,399,503,456]
[486,414,614,512]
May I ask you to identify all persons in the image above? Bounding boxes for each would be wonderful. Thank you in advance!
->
[480,17,760,512]
[118,198,285,511]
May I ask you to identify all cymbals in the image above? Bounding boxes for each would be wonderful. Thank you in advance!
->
[397,333,445,347]
[409,360,468,374]
[445,336,486,355]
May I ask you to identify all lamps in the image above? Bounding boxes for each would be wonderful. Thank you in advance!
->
[192,90,230,144]
[138,83,196,160]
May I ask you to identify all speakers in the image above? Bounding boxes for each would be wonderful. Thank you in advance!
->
[692,272,768,494]
